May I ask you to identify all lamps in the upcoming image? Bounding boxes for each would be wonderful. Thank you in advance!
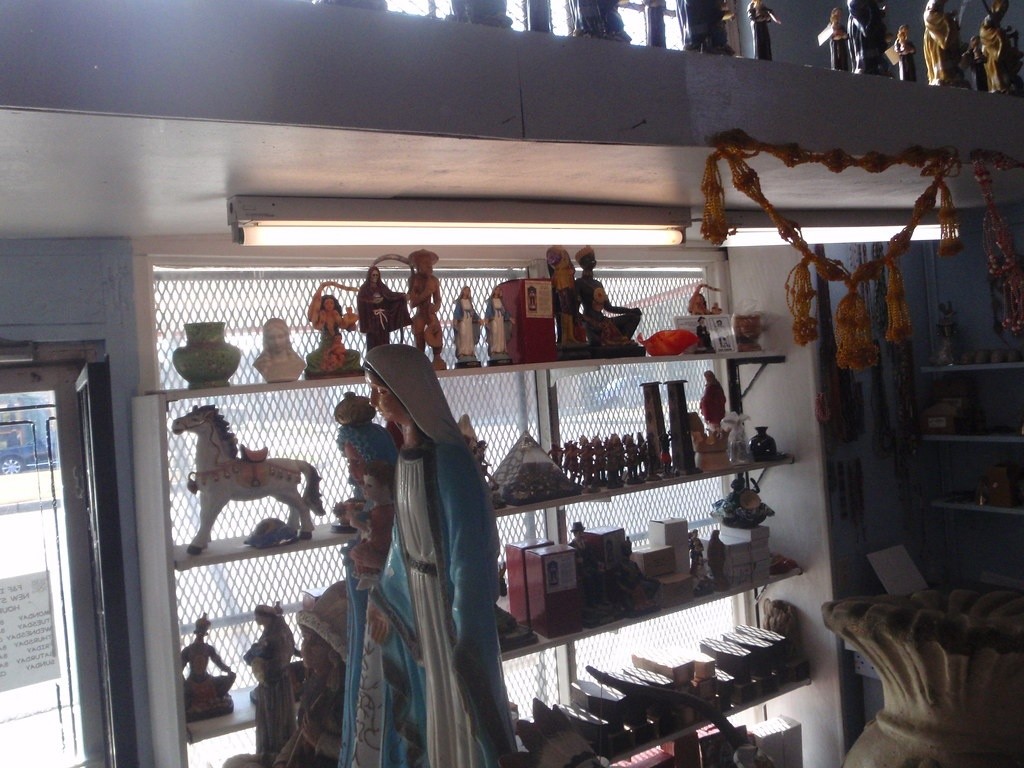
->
[229,193,691,246]
[713,213,964,248]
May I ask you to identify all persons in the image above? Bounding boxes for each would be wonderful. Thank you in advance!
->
[546,431,676,493]
[252,245,645,381]
[687,529,727,596]
[448,0,1024,96]
[178,344,523,768]
[567,523,662,628]
[700,371,727,428]
[697,316,716,353]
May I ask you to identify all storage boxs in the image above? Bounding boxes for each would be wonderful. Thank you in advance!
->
[648,516,690,573]
[525,544,584,639]
[496,278,558,365]
[504,537,555,632]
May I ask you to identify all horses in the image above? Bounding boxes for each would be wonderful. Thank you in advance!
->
[172,405,326,555]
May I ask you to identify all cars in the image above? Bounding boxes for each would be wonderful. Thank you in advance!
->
[0,437,62,475]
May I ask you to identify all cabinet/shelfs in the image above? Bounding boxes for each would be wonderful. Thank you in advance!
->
[922,354,1024,514]
[164,345,812,768]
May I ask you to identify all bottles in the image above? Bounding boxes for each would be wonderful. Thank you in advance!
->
[748,427,777,458]
